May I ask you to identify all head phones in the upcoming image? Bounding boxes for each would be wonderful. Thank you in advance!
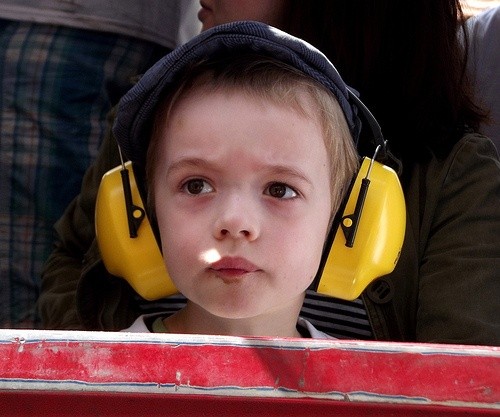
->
[94,85,407,304]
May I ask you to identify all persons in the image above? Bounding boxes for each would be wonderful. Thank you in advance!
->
[0,0,202,331]
[457,4,500,138]
[38,0,500,348]
[115,20,365,340]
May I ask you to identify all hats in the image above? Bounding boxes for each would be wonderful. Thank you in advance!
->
[112,21,362,164]
